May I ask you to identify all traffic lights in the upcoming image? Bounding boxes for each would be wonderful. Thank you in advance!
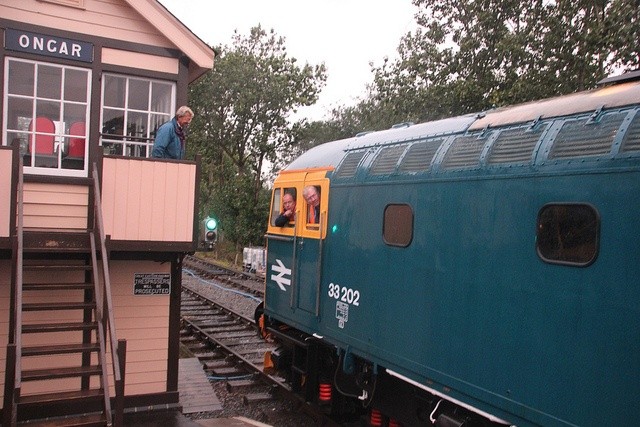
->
[201,217,219,250]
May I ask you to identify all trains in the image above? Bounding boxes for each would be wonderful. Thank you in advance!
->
[253,69,640,427]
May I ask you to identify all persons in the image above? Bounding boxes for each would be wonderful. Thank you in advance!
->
[276,193,296,227]
[303,186,320,225]
[152,105,194,160]
